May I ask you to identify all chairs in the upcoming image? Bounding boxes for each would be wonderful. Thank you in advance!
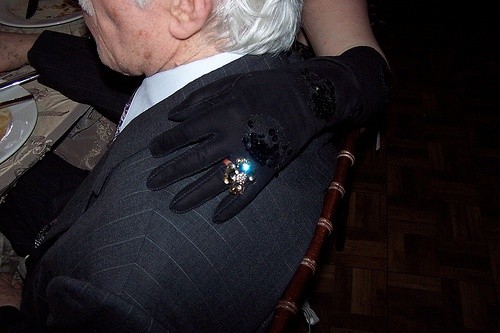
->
[264,125,364,333]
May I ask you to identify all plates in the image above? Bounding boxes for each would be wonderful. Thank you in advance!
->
[0,79,38,164]
[0,0,82,27]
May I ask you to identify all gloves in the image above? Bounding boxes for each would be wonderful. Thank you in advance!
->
[146,46,392,226]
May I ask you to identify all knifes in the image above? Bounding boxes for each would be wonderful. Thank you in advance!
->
[26,0,39,19]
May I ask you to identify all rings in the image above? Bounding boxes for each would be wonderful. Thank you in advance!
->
[224,156,258,197]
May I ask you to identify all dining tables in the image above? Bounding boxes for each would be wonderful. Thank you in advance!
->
[0,17,95,211]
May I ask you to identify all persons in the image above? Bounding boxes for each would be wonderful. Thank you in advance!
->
[0,0,359,333]
[144,0,393,226]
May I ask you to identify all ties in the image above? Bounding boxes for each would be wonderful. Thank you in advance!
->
[111,86,139,141]
